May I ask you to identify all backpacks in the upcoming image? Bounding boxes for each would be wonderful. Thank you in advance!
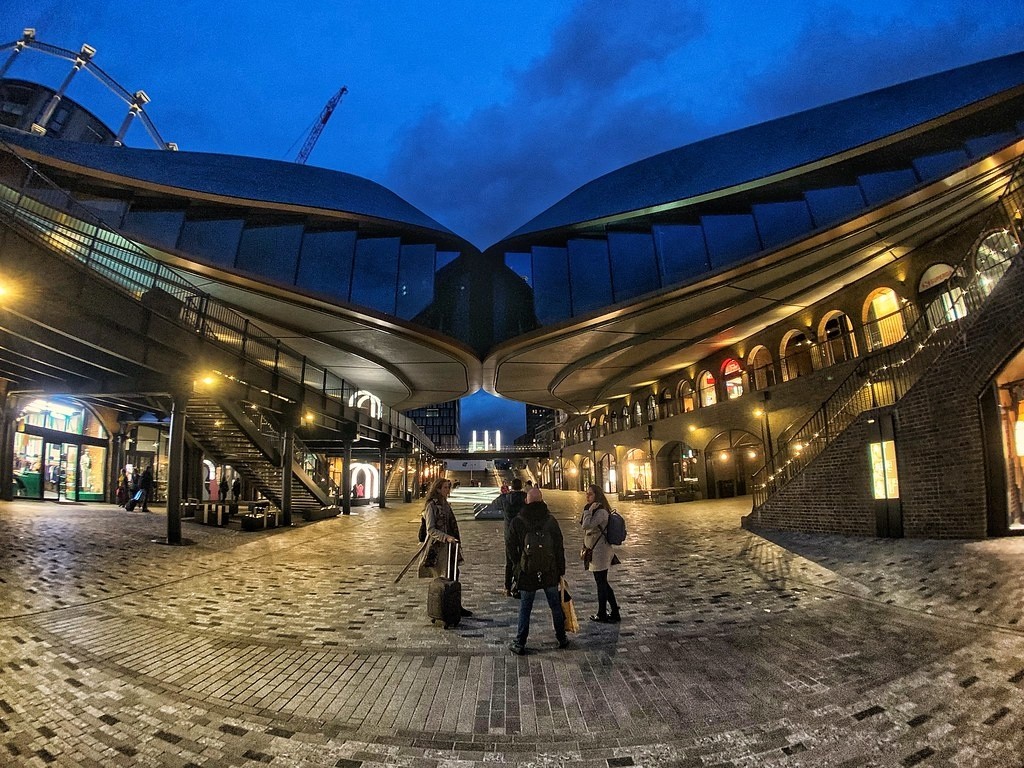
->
[592,504,626,545]
[138,477,145,489]
[514,514,557,591]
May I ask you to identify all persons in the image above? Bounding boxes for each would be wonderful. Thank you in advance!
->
[580,484,622,624]
[353,483,363,498]
[232,478,240,504]
[501,481,509,494]
[417,478,473,617]
[118,466,156,513]
[523,480,532,493]
[493,479,528,597]
[506,489,570,654]
[80,449,92,488]
[220,477,229,505]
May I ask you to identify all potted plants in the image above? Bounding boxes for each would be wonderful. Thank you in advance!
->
[692,484,703,499]
[667,491,676,503]
[674,480,691,502]
[659,490,668,505]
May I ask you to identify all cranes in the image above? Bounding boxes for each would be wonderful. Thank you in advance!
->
[295,85,348,164]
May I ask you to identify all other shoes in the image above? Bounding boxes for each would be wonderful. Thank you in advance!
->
[461,606,473,617]
[590,611,608,622]
[556,636,571,648]
[509,643,526,655]
[142,508,149,512]
[507,588,511,596]
[607,611,621,623]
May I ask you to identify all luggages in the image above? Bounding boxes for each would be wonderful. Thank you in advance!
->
[427,539,461,629]
[125,490,142,511]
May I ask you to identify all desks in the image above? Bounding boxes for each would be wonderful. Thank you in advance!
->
[632,488,663,503]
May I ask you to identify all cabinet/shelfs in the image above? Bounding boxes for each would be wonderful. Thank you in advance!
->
[58,468,84,493]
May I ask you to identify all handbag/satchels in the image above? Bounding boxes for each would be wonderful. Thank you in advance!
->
[580,546,592,570]
[418,517,426,542]
[559,576,579,633]
[611,554,621,565]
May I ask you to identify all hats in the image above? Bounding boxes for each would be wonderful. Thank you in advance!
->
[525,480,532,485]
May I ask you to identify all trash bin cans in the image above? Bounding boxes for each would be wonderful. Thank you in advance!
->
[716,479,735,498]
[407,488,412,503]
